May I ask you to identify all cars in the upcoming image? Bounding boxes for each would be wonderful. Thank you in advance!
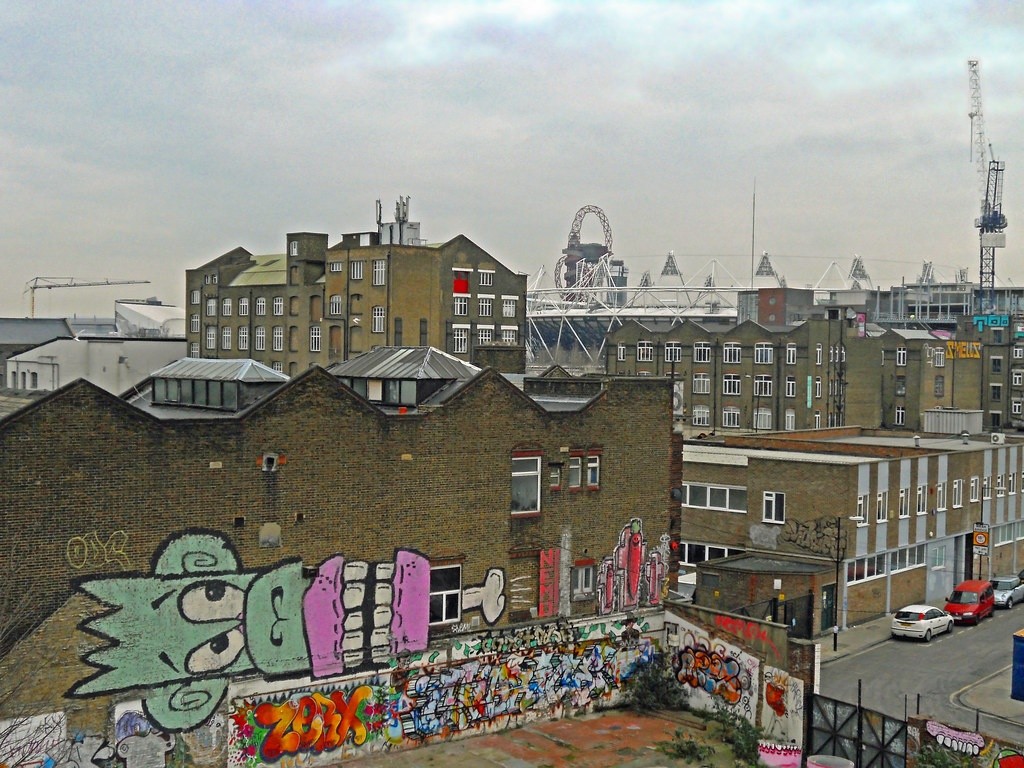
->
[992,576,1024,608]
[890,605,954,643]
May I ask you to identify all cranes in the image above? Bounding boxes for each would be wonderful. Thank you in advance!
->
[23,276,152,319]
[965,58,1007,317]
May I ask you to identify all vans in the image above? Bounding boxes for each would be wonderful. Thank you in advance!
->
[944,581,995,623]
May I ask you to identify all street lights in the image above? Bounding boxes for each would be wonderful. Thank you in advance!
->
[978,486,1008,579]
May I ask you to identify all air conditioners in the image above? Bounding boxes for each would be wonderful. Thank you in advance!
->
[990,433,1005,445]
